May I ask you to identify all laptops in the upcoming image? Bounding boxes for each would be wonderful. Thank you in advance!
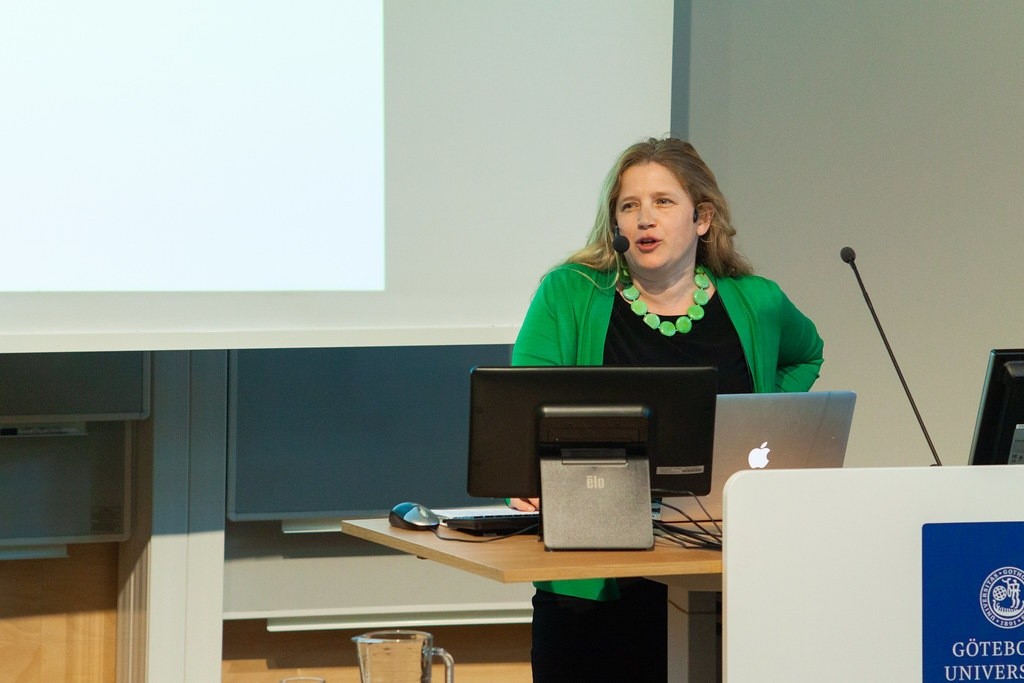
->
[651,388,859,535]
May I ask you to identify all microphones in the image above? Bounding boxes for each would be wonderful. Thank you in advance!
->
[611,218,630,254]
[839,246,943,468]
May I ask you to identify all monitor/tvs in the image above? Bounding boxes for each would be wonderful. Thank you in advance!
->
[968,349,1024,466]
[465,365,717,551]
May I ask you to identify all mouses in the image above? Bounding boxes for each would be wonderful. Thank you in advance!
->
[388,502,439,532]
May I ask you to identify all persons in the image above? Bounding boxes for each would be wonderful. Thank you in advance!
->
[504,132,825,682]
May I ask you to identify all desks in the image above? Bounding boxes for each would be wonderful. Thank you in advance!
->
[341,504,724,683]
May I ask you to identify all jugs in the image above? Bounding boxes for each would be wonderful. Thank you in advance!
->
[350,629,454,683]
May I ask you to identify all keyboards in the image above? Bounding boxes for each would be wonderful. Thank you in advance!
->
[442,513,540,537]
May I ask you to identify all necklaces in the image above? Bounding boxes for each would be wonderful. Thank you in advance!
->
[616,255,712,338]
